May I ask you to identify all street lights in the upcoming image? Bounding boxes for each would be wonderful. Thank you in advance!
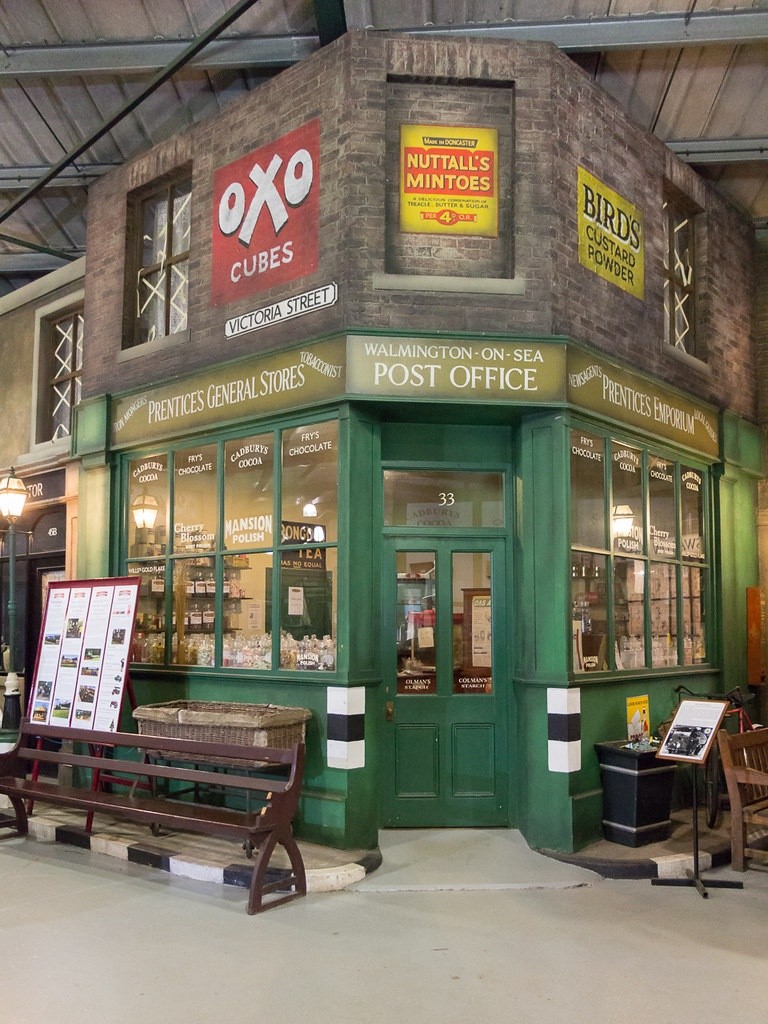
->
[610,504,637,659]
[0,466,30,738]
[132,487,159,639]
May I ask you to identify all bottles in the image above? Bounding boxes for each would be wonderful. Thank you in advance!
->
[145,525,245,629]
[572,601,592,634]
[621,636,692,667]
[641,707,649,739]
[129,631,337,672]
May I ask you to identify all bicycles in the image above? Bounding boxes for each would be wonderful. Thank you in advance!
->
[675,686,767,829]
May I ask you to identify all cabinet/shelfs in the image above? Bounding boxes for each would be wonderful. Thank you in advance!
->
[125,561,253,631]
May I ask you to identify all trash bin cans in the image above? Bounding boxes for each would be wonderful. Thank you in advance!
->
[592,738,678,848]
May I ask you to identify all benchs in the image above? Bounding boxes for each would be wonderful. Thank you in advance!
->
[0,717,306,915]
[718,729,768,872]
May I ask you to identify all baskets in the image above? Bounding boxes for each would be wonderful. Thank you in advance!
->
[132,700,312,771]
